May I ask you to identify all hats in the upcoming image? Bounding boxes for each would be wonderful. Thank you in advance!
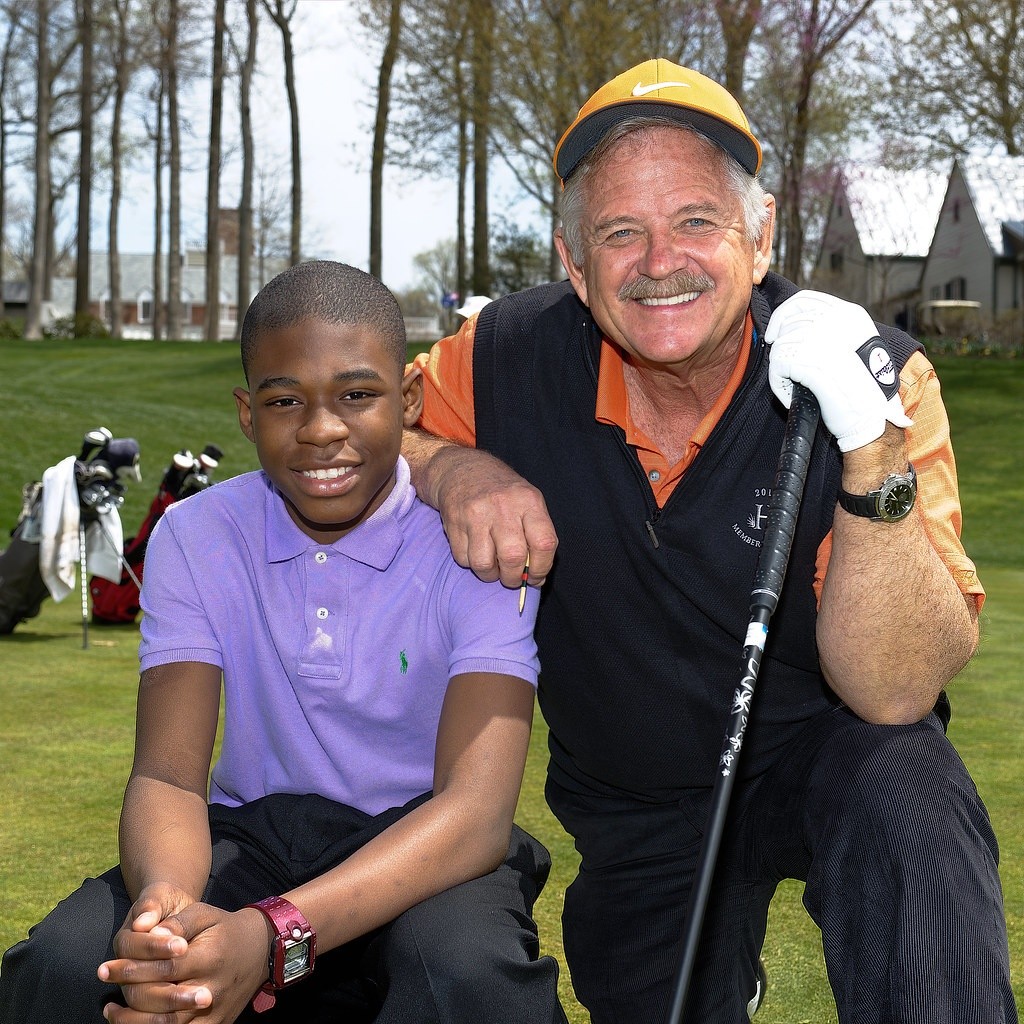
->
[553,56,762,185]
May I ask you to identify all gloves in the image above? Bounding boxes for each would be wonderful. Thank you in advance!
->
[764,291,912,454]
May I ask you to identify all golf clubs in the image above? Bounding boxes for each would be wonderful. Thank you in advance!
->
[0,426,224,650]
[667,380,820,1024]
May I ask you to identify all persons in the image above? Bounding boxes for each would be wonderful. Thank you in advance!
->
[400,58,1018,1024]
[0,261,568,1024]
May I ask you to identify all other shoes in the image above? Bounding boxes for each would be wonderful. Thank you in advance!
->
[744,957,766,1018]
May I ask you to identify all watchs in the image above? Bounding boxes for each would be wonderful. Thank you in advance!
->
[838,463,918,523]
[246,895,316,1012]
